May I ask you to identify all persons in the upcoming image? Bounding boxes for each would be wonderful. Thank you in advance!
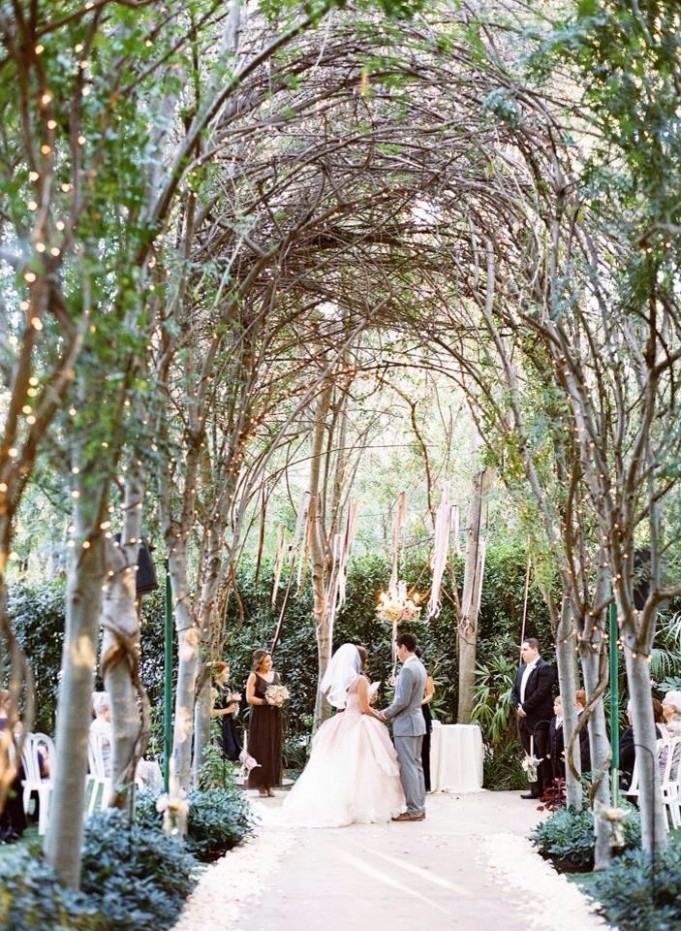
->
[413,645,435,793]
[619,698,636,791]
[245,649,283,799]
[332,653,379,713]
[511,638,557,801]
[575,689,591,774]
[89,691,162,799]
[547,696,569,811]
[282,642,407,828]
[626,698,670,812]
[377,634,428,821]
[660,689,681,784]
[209,661,242,764]
[0,689,25,842]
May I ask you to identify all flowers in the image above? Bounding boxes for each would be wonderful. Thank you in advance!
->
[266,684,292,707]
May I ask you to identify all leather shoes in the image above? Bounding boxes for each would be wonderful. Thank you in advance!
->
[421,812,426,818]
[392,811,423,821]
[520,793,542,799]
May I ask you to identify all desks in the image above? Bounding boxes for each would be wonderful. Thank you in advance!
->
[429,720,483,792]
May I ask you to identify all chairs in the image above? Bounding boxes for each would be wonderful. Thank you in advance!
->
[619,737,681,833]
[14,715,158,837]
